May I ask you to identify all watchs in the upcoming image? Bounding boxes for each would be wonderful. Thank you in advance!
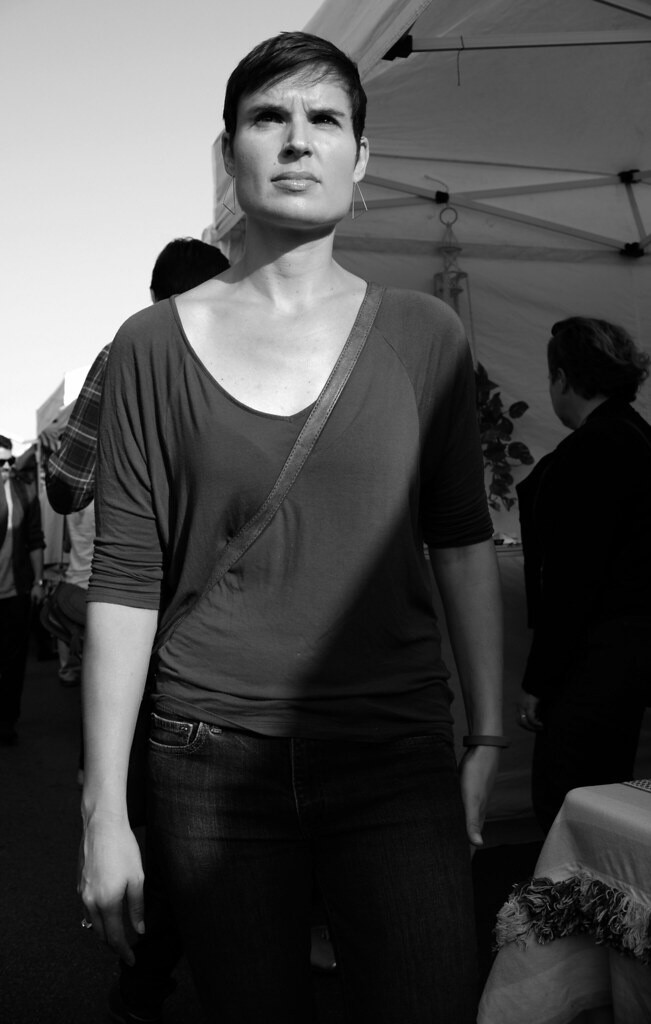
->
[33,580,43,586]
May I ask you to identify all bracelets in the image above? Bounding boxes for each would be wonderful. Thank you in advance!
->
[458,736,507,749]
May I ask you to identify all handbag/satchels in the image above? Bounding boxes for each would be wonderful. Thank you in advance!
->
[39,580,89,655]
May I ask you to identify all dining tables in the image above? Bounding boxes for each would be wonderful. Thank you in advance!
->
[477,779,650,1024]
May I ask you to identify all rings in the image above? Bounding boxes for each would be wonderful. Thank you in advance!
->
[522,715,527,718]
[81,917,92,929]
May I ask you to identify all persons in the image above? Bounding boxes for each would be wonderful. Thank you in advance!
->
[77,31,510,1023]
[516,316,651,840]
[48,237,231,513]
[0,435,46,671]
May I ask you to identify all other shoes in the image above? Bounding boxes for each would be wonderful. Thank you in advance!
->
[1,725,21,751]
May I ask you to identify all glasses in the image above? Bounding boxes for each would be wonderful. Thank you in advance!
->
[1,457,16,468]
